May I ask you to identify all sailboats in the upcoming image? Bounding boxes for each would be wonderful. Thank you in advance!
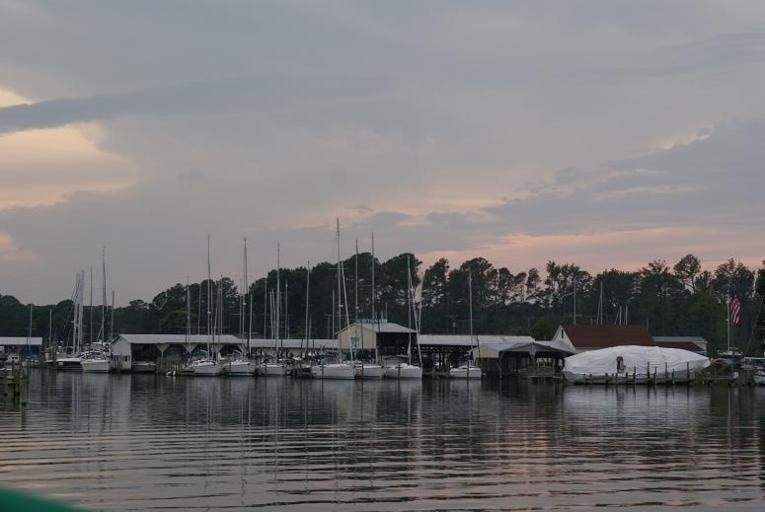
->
[190,375,425,505]
[27,370,114,502]
[721,385,745,453]
[712,288,745,359]
[451,380,483,479]
[740,356,765,385]
[450,266,481,379]
[752,386,765,400]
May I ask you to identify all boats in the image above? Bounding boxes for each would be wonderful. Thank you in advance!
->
[23,245,115,372]
[4,356,23,365]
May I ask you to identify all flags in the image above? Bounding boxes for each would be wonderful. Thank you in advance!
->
[729,295,742,327]
